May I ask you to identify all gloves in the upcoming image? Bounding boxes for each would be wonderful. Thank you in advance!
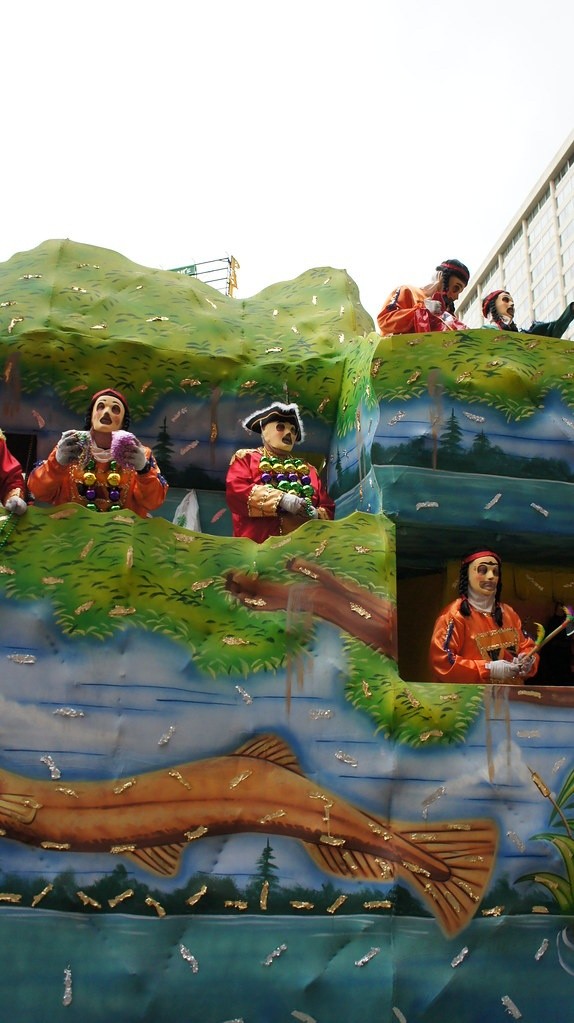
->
[6,496,27,515]
[55,430,81,466]
[489,660,519,681]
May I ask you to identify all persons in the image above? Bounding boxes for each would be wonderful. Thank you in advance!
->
[225,402,334,544]
[482,290,574,339]
[377,259,470,333]
[25,389,168,517]
[0,430,27,516]
[427,547,539,686]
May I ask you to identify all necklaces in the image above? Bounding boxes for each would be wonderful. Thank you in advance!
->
[84,456,120,511]
[0,510,19,550]
[259,448,314,512]
